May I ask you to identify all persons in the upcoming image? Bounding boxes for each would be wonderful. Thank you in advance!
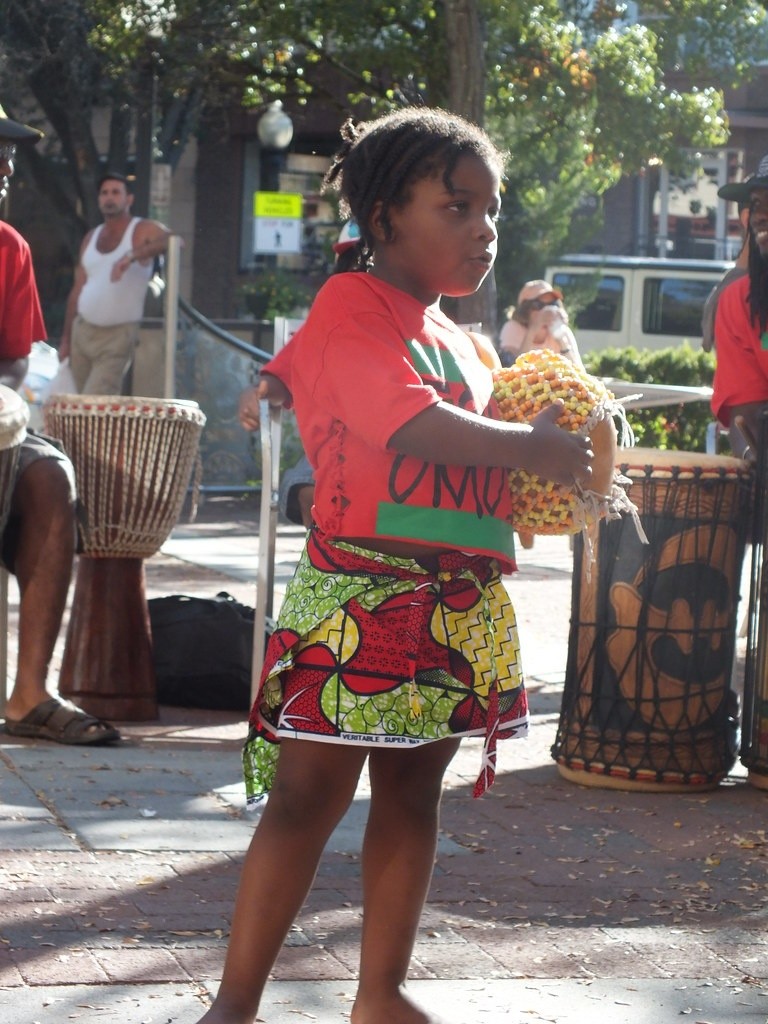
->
[500,279,584,373]
[238,218,371,429]
[0,106,122,744]
[701,156,768,460]
[196,108,594,1024]
[56,172,185,396]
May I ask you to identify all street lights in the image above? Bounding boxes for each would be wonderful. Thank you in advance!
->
[255,98,295,277]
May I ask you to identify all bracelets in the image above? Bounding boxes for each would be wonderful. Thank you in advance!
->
[125,252,135,262]
[742,446,749,460]
[560,347,570,354]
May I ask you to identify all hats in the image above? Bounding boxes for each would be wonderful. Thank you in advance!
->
[519,280,552,307]
[717,157,768,201]
[0,105,44,146]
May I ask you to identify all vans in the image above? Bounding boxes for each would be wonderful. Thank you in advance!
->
[543,253,736,364]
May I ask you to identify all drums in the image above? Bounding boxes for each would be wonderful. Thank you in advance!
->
[549,445,754,796]
[740,548,767,792]
[42,392,208,729]
[0,386,32,541]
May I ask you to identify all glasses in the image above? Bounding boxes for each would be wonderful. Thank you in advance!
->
[525,298,563,312]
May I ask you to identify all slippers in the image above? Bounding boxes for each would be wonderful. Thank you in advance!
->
[3,698,120,746]
